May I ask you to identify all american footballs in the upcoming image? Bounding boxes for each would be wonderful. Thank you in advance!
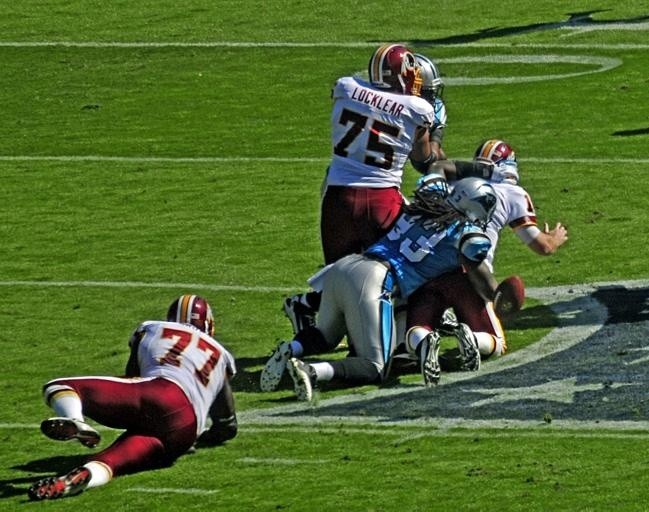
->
[493,276,524,319]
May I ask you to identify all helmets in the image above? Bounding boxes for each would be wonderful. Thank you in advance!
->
[472,138,517,170]
[444,176,498,225]
[166,294,215,338]
[368,43,416,96]
[406,53,443,92]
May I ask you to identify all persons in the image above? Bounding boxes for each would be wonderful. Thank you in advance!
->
[259,157,505,403]
[400,138,568,389]
[25,292,237,503]
[319,38,460,374]
[284,52,448,338]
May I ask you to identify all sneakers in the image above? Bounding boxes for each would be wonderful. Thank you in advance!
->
[282,293,326,346]
[40,416,102,448]
[260,339,294,393]
[421,332,442,392]
[27,466,92,502]
[286,358,316,403]
[453,323,481,373]
